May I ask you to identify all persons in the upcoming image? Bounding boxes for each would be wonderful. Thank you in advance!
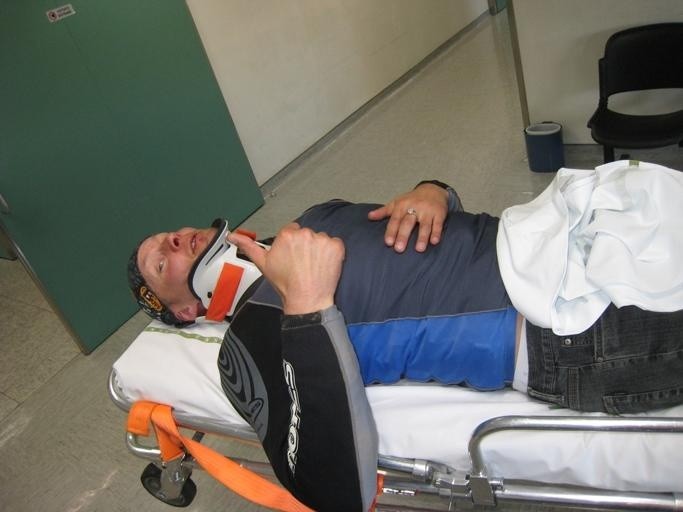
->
[125,178,683,511]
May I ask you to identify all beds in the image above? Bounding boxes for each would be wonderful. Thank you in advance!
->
[109,317,683,511]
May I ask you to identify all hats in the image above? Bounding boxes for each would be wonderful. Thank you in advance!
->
[128,232,196,329]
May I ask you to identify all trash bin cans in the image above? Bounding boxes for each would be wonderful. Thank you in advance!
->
[521,120,565,174]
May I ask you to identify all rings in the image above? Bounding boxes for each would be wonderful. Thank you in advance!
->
[405,207,418,217]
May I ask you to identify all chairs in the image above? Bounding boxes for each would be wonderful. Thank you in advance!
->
[588,22,683,163]
